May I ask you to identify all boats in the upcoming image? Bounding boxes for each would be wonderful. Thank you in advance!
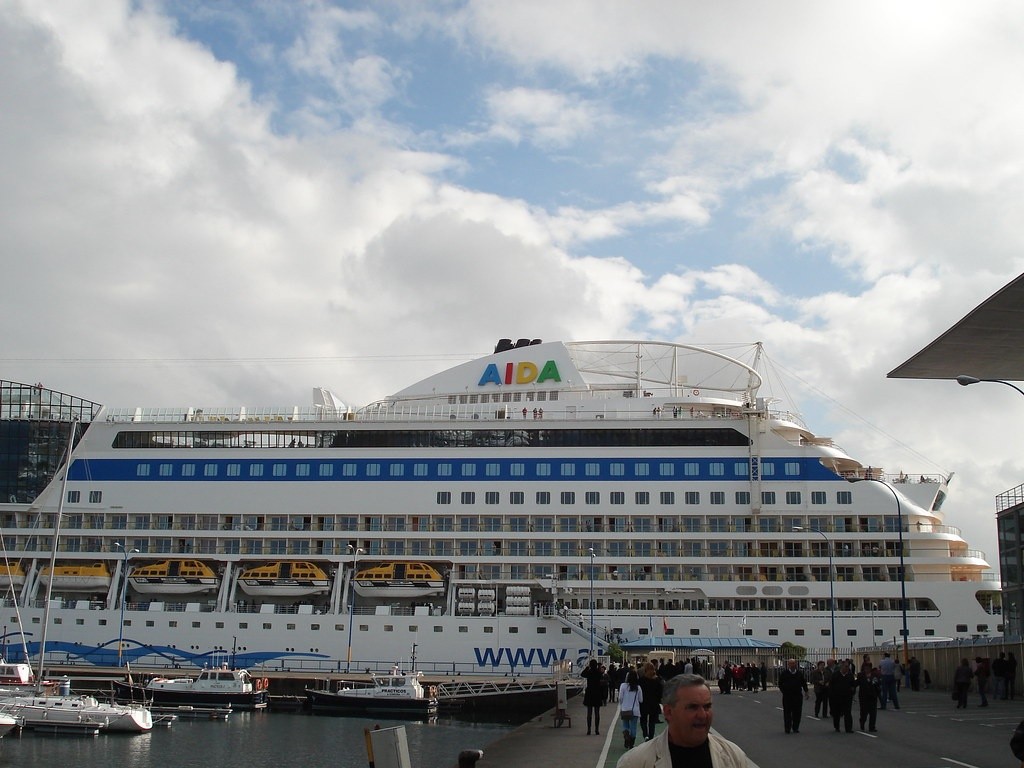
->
[304,640,443,710]
[96,635,269,710]
[237,558,331,597]
[350,562,445,598]
[129,557,220,595]
[0,710,17,737]
[37,561,112,593]
[1,560,27,591]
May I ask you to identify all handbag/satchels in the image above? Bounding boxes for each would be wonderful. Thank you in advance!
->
[952,682,960,700]
[900,665,904,673]
[621,711,633,720]
[751,677,754,681]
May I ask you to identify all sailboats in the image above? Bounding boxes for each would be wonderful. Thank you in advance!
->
[0,411,156,732]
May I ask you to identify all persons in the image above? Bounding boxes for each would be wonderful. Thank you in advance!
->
[562,605,569,618]
[618,670,643,749]
[950,651,1018,708]
[638,663,664,742]
[604,626,614,643]
[577,612,584,629]
[812,652,922,734]
[778,658,810,734]
[716,659,768,695]
[616,673,760,768]
[606,654,711,724]
[580,659,609,736]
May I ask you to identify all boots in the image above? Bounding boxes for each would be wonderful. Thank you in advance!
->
[629,735,636,749]
[623,730,630,748]
[595,718,599,735]
[587,717,591,735]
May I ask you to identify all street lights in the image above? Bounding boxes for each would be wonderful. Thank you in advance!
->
[114,541,140,666]
[346,543,364,673]
[588,547,597,658]
[793,525,837,662]
[847,476,911,689]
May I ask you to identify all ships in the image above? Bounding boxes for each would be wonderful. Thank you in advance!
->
[0,336,1006,699]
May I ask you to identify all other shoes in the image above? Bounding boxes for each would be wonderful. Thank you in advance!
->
[978,702,988,707]
[655,720,664,724]
[869,729,877,732]
[861,729,864,732]
[835,728,840,732]
[957,704,966,708]
[785,729,790,734]
[793,729,798,733]
[846,730,854,733]
[644,737,649,742]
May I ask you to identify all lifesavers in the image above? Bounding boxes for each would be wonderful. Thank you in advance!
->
[257,679,263,691]
[264,678,269,688]
